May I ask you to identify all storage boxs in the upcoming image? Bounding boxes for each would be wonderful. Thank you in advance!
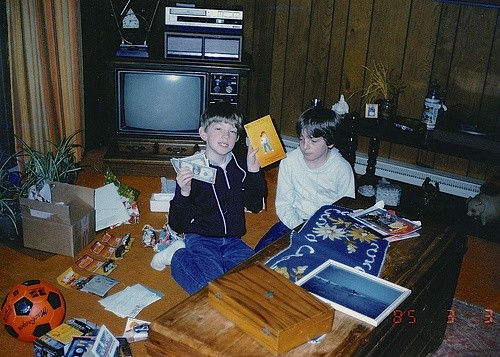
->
[208,263,336,357]
[33,318,133,357]
[149,193,175,212]
[19,182,95,258]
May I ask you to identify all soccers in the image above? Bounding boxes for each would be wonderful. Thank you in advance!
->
[2,278,68,343]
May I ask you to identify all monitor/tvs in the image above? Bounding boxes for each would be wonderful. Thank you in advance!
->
[113,62,251,141]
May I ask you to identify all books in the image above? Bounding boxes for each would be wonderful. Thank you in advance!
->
[81,275,120,297]
[351,207,422,242]
[244,114,287,168]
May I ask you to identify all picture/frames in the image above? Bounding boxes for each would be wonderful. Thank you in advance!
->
[363,102,381,121]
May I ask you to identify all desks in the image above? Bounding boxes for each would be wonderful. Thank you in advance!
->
[347,111,500,236]
[144,197,468,357]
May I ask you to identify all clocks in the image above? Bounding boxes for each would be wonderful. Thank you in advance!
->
[108,0,160,58]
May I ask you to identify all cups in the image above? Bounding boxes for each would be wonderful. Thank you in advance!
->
[421,89,441,131]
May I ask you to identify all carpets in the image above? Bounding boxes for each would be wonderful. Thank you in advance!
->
[427,299,500,357]
[0,238,56,262]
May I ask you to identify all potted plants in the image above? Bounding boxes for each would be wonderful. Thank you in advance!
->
[0,128,101,242]
[346,56,407,122]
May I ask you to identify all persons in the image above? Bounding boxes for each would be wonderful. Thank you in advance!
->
[351,287,359,299]
[253,105,355,254]
[150,101,269,297]
[318,277,333,286]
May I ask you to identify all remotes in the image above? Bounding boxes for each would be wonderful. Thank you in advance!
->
[176,3,195,8]
